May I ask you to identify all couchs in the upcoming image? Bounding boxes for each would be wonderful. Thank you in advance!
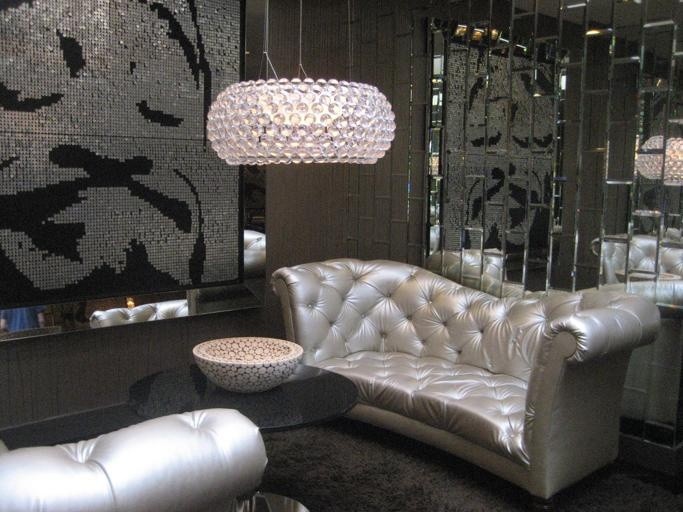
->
[242,227,267,280]
[89,297,188,329]
[427,247,682,444]
[270,255,663,512]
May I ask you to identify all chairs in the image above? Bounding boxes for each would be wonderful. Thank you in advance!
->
[595,235,683,289]
[1,404,269,512]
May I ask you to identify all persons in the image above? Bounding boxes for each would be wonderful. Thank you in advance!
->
[0,305,49,333]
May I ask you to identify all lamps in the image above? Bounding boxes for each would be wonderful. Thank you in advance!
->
[206,0,398,168]
[636,136,683,185]
